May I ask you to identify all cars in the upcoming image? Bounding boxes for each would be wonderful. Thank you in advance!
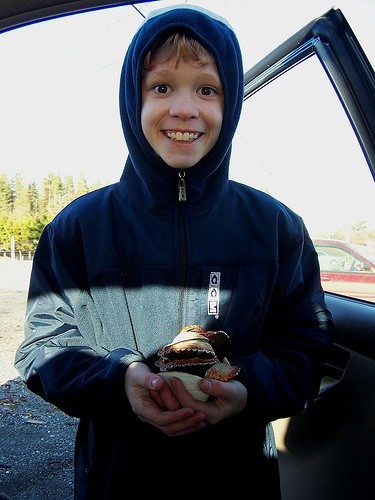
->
[308,238,375,306]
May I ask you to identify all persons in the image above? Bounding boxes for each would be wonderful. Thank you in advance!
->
[14,3,334,500]
